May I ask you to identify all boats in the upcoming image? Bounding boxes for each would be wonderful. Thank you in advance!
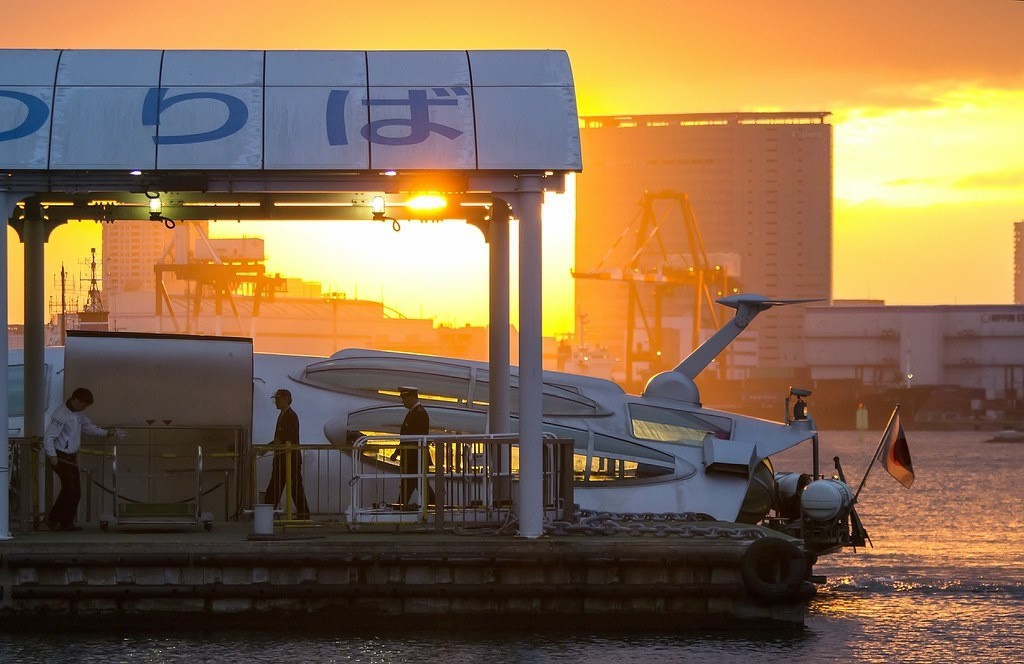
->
[8,293,916,557]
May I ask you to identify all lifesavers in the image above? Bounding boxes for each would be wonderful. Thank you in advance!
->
[735,536,808,603]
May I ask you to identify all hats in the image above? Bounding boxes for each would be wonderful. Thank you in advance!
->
[397,385,417,396]
[269,389,291,399]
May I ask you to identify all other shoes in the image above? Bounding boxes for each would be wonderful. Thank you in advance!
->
[292,514,308,519]
[61,523,84,531]
[43,517,60,530]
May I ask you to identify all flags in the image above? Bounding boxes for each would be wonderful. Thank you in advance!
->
[877,413,914,490]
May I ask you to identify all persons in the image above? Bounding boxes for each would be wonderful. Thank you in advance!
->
[388,386,435,510]
[258,389,310,520]
[42,387,131,533]
